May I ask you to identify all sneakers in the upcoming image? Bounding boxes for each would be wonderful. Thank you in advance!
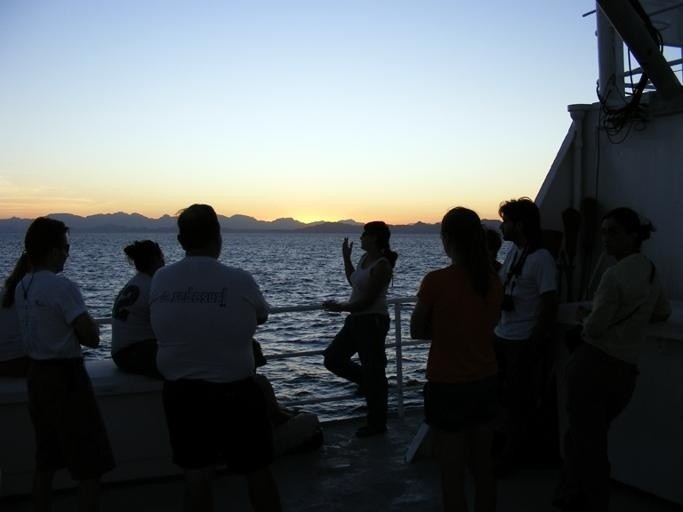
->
[356,423,386,437]
[355,383,364,397]
[71,468,115,487]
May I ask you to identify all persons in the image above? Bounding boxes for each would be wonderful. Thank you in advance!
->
[149,205,284,512]
[0,252,30,378]
[113,241,165,378]
[13,217,117,511]
[324,222,392,437]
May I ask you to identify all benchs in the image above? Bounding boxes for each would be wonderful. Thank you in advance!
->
[2,367,174,496]
